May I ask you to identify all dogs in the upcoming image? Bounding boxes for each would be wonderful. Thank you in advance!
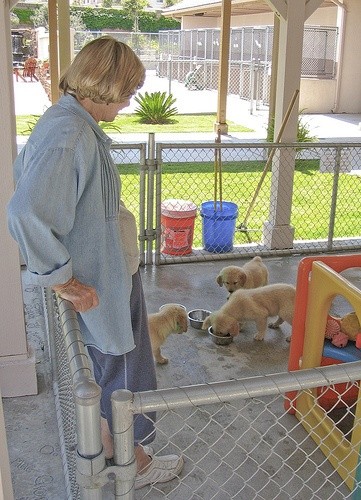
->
[147,303,192,365]
[200,282,296,344]
[215,255,270,302]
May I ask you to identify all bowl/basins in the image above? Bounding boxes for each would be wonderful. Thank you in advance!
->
[208,325,233,345]
[187,308,212,328]
[159,304,186,311]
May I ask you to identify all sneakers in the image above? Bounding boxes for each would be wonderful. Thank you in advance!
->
[134,454,183,489]
[143,446,154,454]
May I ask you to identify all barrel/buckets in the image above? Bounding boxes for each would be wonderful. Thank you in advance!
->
[200,201,238,254]
[161,199,197,255]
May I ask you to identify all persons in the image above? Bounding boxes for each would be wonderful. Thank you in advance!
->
[24,52,33,77]
[7,35,183,489]
